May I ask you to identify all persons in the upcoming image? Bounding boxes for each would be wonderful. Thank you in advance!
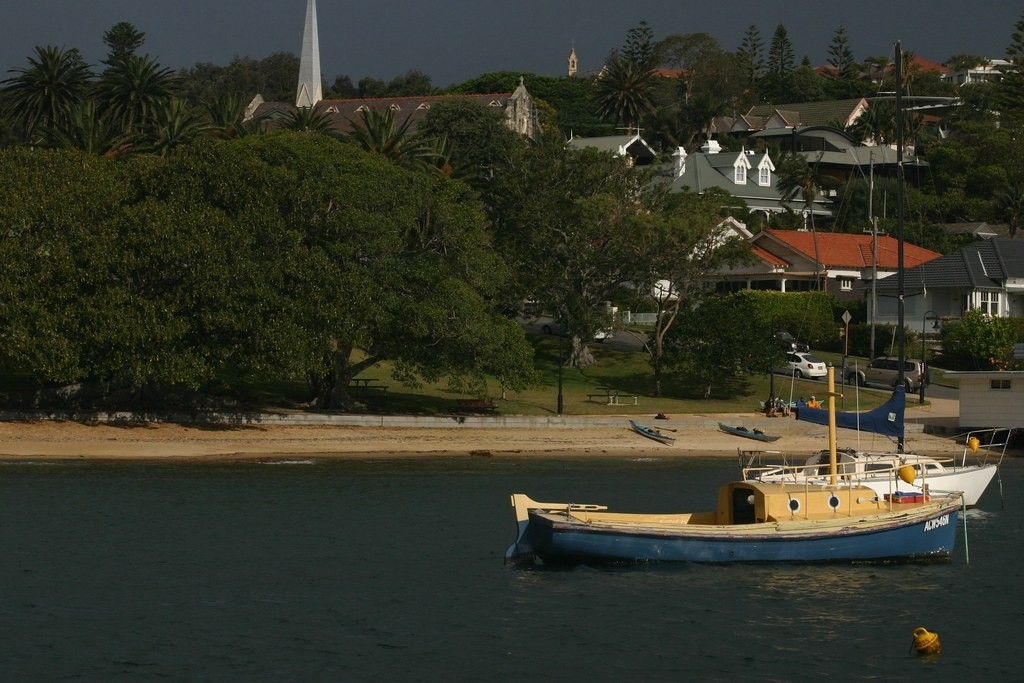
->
[764,396,822,417]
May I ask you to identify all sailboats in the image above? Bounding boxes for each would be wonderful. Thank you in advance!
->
[739,37,1013,511]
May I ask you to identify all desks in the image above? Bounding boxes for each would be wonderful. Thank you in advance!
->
[596,387,626,402]
[351,379,379,393]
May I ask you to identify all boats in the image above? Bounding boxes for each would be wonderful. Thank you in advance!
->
[628,418,678,445]
[503,361,964,569]
[717,420,783,442]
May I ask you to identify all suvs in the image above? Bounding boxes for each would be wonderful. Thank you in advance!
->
[840,356,922,394]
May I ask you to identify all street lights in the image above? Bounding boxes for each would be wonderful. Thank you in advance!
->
[919,310,942,404]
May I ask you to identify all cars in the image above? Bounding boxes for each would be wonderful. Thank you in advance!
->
[542,312,612,344]
[772,331,810,353]
[780,351,828,380]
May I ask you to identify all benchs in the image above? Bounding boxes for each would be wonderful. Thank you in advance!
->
[457,399,499,413]
[587,394,633,402]
[347,386,389,395]
